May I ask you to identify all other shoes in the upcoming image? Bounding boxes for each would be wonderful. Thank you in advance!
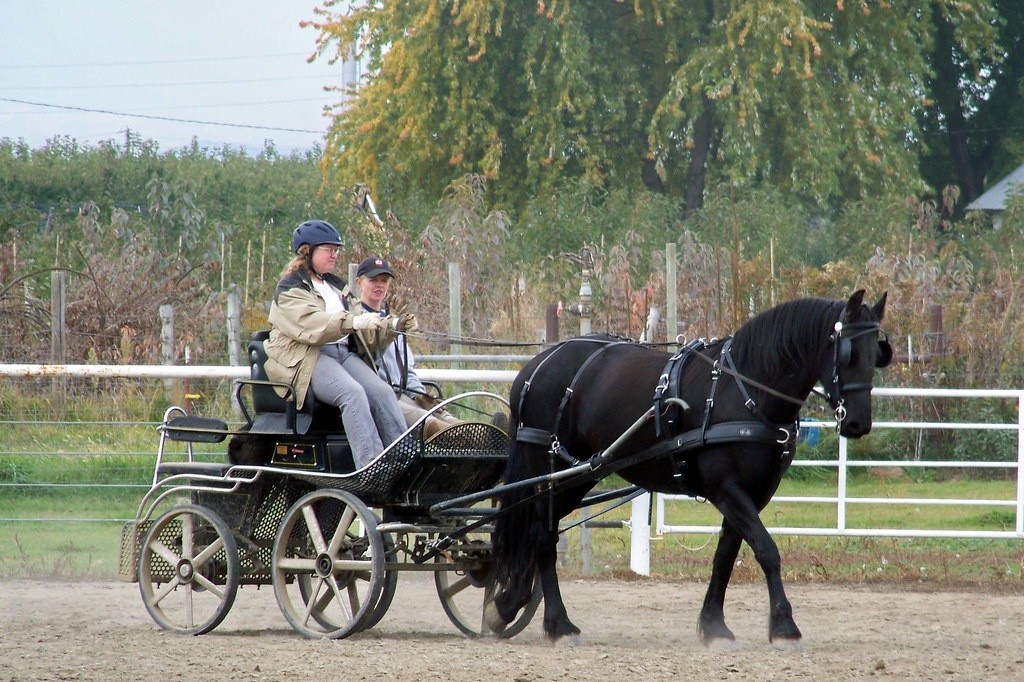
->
[488,410,513,451]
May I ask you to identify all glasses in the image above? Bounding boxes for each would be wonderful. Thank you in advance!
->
[318,246,341,253]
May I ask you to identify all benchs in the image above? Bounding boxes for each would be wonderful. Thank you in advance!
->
[249,330,345,429]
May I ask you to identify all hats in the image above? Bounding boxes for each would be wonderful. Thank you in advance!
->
[357,257,396,280]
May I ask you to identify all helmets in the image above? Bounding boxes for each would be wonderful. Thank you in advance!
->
[291,219,344,253]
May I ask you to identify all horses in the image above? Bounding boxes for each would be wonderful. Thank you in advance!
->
[483,287,888,651]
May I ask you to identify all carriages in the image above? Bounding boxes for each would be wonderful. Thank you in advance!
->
[115,291,894,661]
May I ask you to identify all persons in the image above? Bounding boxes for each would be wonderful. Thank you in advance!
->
[355,257,510,451]
[263,220,417,490]
